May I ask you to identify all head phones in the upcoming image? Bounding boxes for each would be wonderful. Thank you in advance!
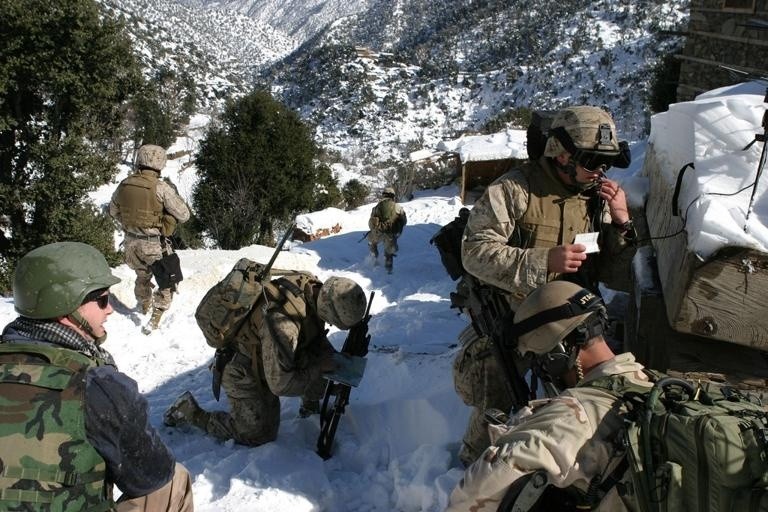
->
[534,341,570,380]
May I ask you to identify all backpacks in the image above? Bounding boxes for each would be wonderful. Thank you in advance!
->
[581,367,768,512]
[195,258,317,350]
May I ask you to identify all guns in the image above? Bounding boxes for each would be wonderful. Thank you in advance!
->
[357,231,371,244]
[316,290,375,460]
[429,208,531,414]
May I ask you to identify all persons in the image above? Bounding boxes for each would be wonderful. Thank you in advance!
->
[459,105,638,466]
[165,276,367,448]
[367,187,407,273]
[0,242,193,512]
[445,281,767,512]
[110,145,190,333]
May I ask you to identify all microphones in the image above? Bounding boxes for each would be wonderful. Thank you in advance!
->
[594,170,609,184]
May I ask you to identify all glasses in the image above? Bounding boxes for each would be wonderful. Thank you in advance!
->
[554,125,623,173]
[90,293,108,309]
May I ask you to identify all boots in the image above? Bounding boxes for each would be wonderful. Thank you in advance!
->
[162,391,211,433]
[139,301,163,335]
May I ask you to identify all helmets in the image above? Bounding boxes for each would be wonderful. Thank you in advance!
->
[13,241,122,320]
[512,280,607,359]
[383,187,395,197]
[316,275,366,331]
[544,105,620,158]
[136,144,167,171]
